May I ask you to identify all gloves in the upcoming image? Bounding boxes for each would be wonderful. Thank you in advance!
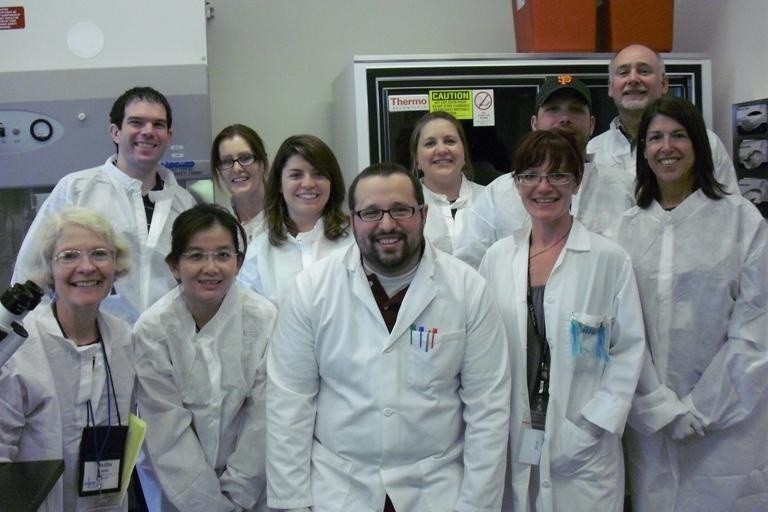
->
[672,412,707,443]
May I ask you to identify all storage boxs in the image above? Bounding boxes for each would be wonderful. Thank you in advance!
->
[512,0,598,53]
[598,0,674,52]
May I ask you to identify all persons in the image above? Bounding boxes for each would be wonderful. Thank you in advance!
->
[448,74,632,272]
[266,163,512,512]
[210,124,277,249]
[476,130,646,511]
[11,86,198,328]
[599,95,768,512]
[410,111,486,257]
[235,134,356,308]
[0,208,133,512]
[131,204,276,511]
[586,42,741,196]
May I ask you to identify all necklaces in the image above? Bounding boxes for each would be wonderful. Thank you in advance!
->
[529,227,573,259]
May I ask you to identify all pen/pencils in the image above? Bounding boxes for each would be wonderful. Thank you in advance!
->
[410,323,438,353]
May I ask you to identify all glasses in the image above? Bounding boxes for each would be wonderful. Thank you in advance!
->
[217,154,257,171]
[353,205,423,223]
[182,251,240,262]
[53,247,118,263]
[514,171,573,186]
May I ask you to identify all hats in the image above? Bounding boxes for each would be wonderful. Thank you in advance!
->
[535,74,594,108]
